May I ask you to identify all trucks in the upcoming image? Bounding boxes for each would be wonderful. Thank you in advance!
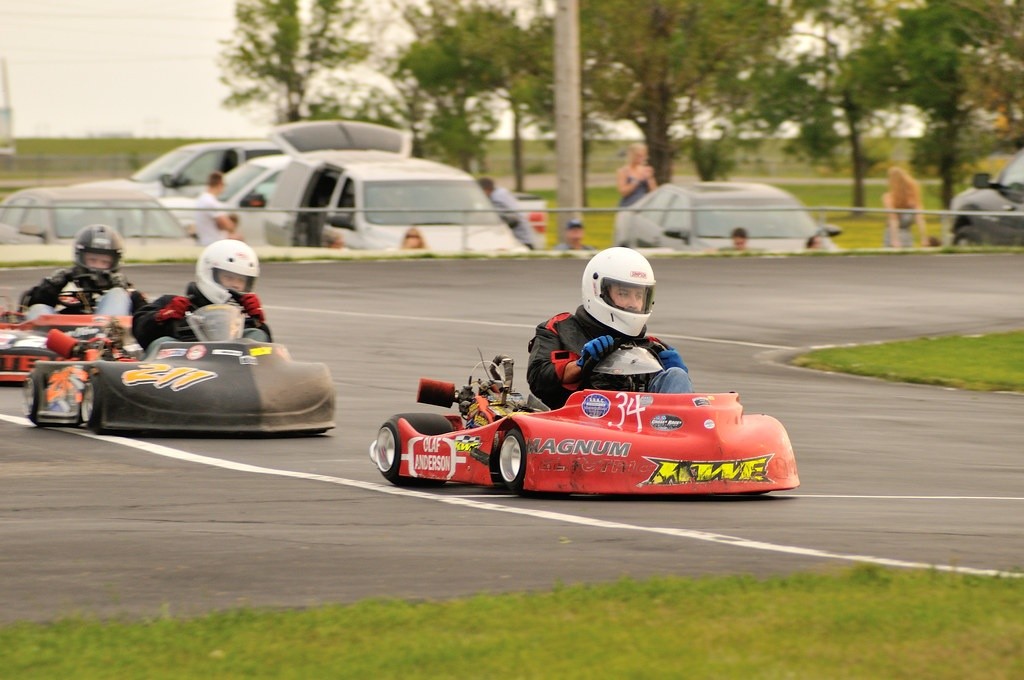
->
[127,122,416,200]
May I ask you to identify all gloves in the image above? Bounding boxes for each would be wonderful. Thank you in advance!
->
[658,346,688,372]
[577,335,614,366]
[109,273,130,289]
[239,293,265,323]
[154,295,196,322]
[45,269,67,286]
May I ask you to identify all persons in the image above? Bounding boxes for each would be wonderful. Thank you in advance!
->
[18,223,148,332]
[195,171,243,246]
[528,247,693,410]
[478,177,534,248]
[555,218,595,250]
[403,226,425,250]
[616,143,656,209]
[133,239,272,355]
[808,232,822,249]
[730,227,747,250]
[884,166,927,250]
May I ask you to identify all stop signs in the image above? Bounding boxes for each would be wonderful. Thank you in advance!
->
[527,212,547,232]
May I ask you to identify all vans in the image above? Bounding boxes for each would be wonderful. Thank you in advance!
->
[268,149,535,256]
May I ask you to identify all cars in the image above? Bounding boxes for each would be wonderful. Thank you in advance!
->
[946,147,1024,248]
[0,184,205,262]
[155,153,296,245]
[612,182,842,252]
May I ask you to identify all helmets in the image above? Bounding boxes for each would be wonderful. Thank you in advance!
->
[195,238,261,303]
[74,223,125,271]
[582,247,657,336]
